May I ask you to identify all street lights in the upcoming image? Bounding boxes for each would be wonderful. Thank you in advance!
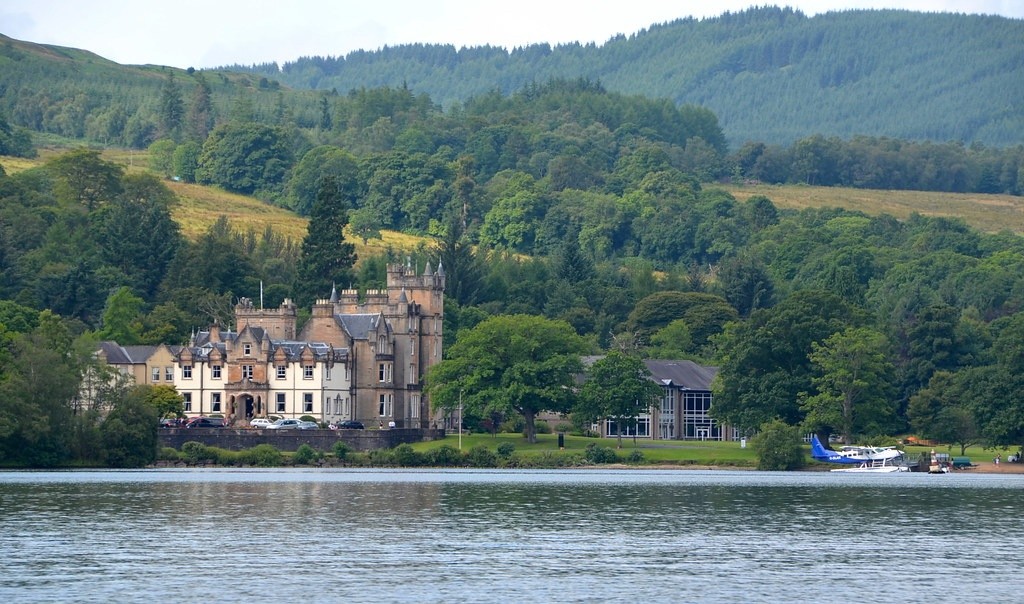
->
[459,388,464,449]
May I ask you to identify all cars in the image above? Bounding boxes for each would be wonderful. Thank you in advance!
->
[335,419,365,429]
[250,418,275,429]
[296,421,320,431]
[161,415,229,428]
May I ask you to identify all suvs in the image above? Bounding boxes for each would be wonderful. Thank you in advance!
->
[265,418,305,430]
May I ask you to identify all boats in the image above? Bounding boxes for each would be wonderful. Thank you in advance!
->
[811,434,910,474]
